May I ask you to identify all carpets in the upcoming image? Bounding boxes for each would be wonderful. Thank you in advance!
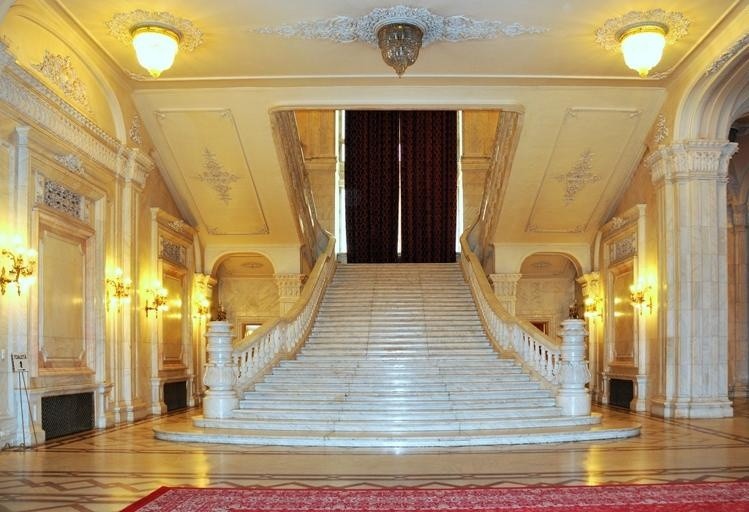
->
[118,479,749,512]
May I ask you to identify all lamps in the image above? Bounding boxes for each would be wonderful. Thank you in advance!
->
[127,19,184,80]
[0,234,38,296]
[582,293,604,320]
[629,275,658,314]
[371,16,428,78]
[105,268,211,322]
[613,21,668,79]
